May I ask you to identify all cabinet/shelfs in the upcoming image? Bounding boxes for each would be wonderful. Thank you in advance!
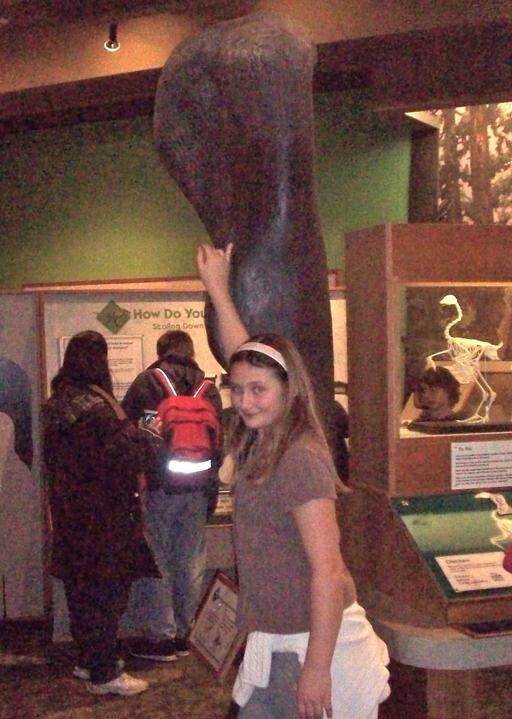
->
[335,219,512,719]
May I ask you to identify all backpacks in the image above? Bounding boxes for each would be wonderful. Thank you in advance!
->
[153,366,221,493]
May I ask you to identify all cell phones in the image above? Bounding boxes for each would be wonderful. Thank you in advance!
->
[143,410,158,425]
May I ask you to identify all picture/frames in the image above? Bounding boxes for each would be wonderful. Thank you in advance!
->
[184,568,247,685]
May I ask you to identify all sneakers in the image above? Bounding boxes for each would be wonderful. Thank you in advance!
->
[127,636,192,663]
[71,653,150,696]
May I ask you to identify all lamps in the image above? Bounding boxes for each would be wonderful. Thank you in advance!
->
[103,22,121,52]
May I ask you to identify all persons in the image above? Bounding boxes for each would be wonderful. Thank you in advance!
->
[408,365,468,420]
[39,329,162,696]
[119,331,224,661]
[198,243,391,718]
